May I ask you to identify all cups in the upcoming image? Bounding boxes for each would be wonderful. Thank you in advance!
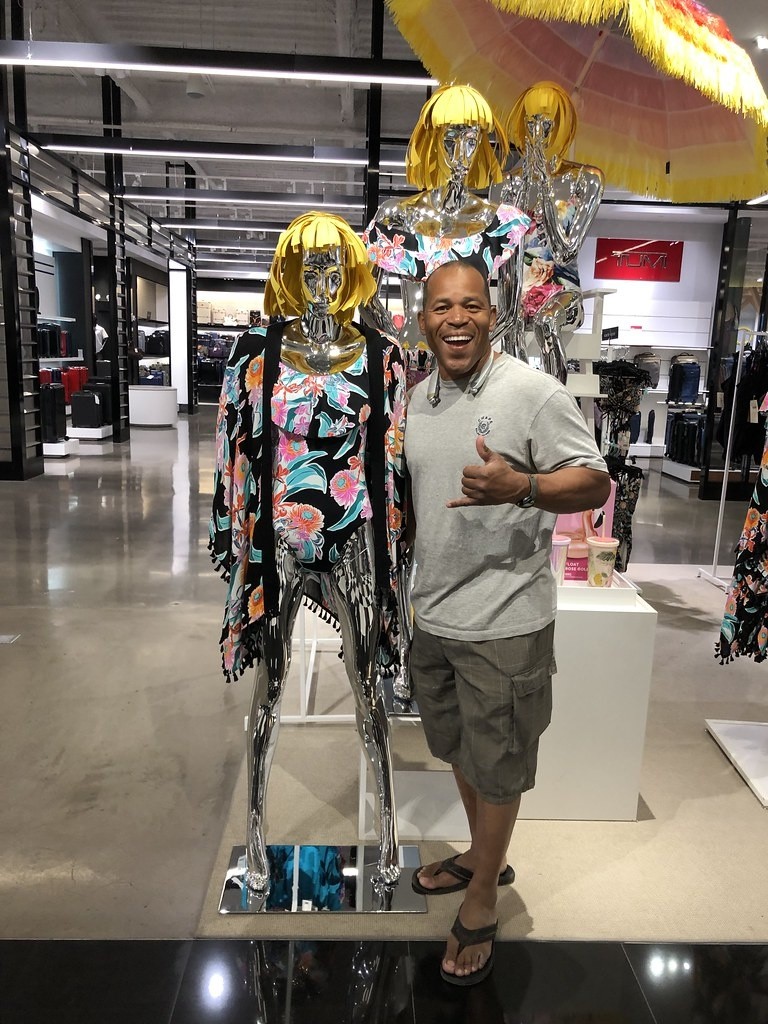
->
[550,534,570,586]
[588,536,619,588]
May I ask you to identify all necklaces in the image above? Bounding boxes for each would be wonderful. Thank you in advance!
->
[425,346,494,405]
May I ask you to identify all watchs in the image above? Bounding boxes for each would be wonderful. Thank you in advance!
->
[516,472,538,509]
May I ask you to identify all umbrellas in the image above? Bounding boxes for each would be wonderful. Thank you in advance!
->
[387,0,768,205]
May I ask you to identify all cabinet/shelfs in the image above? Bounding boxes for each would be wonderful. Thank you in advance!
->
[34,313,234,403]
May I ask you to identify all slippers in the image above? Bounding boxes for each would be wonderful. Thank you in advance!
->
[412,853,515,895]
[440,901,498,986]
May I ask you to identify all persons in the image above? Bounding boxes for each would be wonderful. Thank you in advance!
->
[488,80,604,384]
[362,81,530,701]
[403,263,610,986]
[207,211,406,897]
